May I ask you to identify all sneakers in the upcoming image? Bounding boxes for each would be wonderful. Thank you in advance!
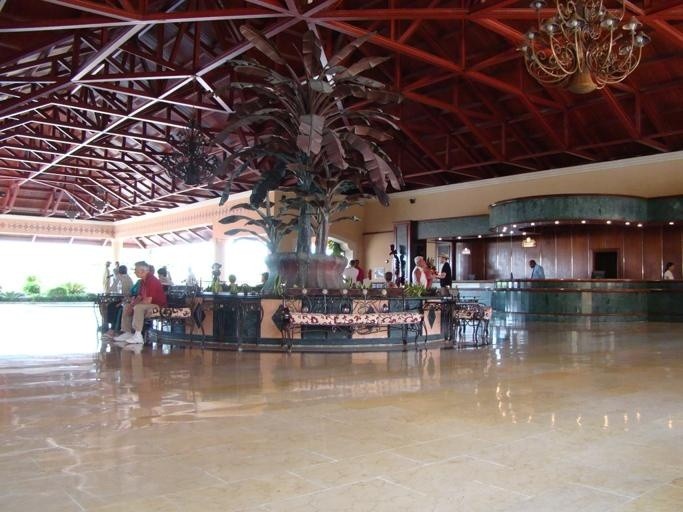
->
[112,332,145,344]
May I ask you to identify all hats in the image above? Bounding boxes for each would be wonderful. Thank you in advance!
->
[436,252,452,259]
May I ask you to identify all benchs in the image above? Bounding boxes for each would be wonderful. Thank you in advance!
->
[279,289,493,356]
[98,296,206,351]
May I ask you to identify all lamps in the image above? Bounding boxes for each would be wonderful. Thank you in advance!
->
[158,72,219,186]
[517,0,652,96]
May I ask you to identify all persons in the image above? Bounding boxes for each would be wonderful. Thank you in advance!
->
[180,266,198,286]
[100,261,174,344]
[412,251,452,296]
[227,275,239,292]
[341,259,372,289]
[381,272,398,288]
[92,342,167,427]
[663,262,675,280]
[528,259,545,282]
[253,271,269,292]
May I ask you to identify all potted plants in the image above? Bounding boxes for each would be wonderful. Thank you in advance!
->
[221,25,406,299]
